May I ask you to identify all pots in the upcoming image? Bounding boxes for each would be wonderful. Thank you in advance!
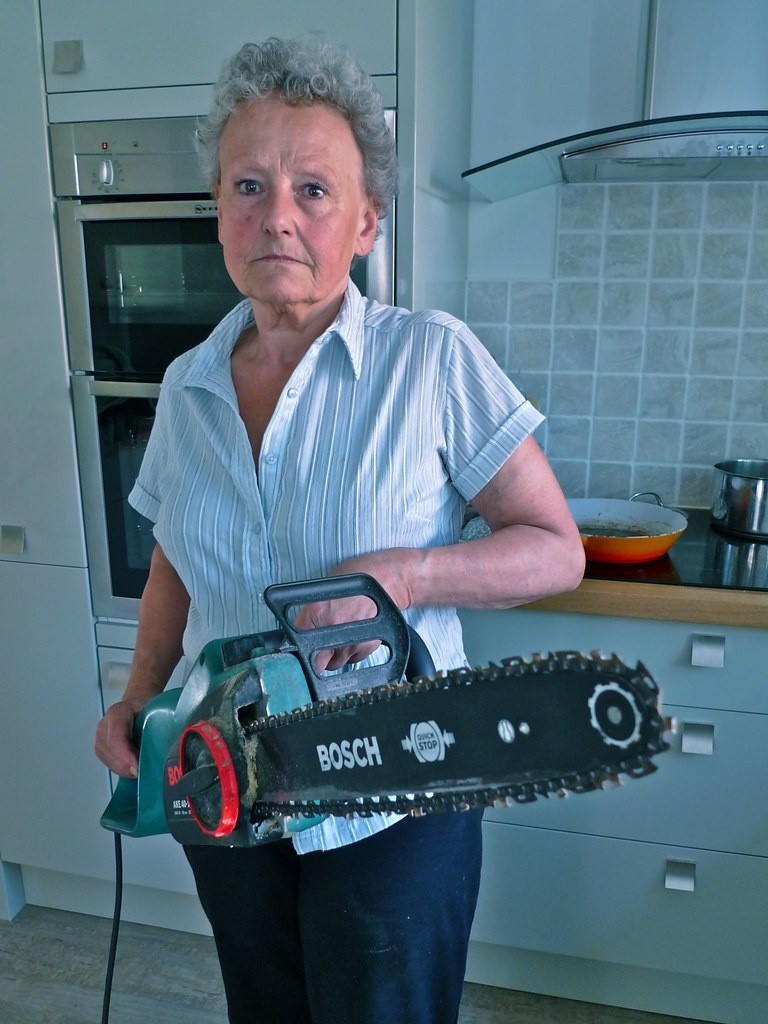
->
[714,458,768,537]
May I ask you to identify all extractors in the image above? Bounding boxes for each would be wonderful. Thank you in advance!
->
[559,115,768,185]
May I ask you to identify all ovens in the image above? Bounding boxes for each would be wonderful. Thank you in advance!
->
[48,109,396,622]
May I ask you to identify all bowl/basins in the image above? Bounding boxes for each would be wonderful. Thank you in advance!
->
[566,498,688,564]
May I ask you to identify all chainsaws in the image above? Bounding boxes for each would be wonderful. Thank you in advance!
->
[100,567,672,848]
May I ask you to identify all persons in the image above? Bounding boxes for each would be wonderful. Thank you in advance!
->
[94,36,586,1024]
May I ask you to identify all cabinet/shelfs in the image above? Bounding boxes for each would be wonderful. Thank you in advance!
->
[0,0,119,883]
[98,645,205,896]
[453,604,768,983]
